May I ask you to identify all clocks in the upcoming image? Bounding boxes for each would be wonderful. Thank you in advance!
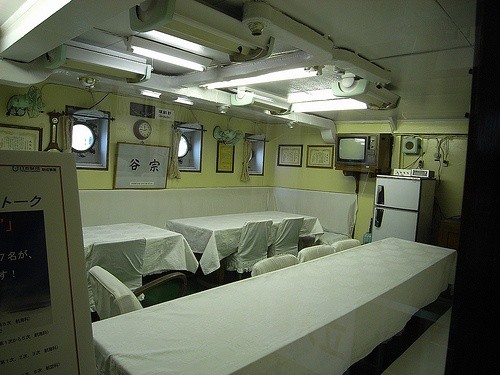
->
[133,120,152,140]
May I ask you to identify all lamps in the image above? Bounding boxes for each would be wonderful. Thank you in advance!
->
[127,36,212,72]
[198,64,325,89]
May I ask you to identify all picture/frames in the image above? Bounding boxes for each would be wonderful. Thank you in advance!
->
[277,144,303,167]
[216,140,235,173]
[0,124,43,151]
[306,145,334,169]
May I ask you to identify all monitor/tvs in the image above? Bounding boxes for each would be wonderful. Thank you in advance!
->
[335,133,392,173]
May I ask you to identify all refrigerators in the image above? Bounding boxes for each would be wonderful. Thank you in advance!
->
[371,175,436,243]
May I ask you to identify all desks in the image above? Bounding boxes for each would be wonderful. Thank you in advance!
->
[167,211,324,293]
[82,223,198,324]
[91,237,458,375]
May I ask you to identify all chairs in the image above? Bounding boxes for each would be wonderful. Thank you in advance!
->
[222,217,360,280]
[88,237,188,320]
